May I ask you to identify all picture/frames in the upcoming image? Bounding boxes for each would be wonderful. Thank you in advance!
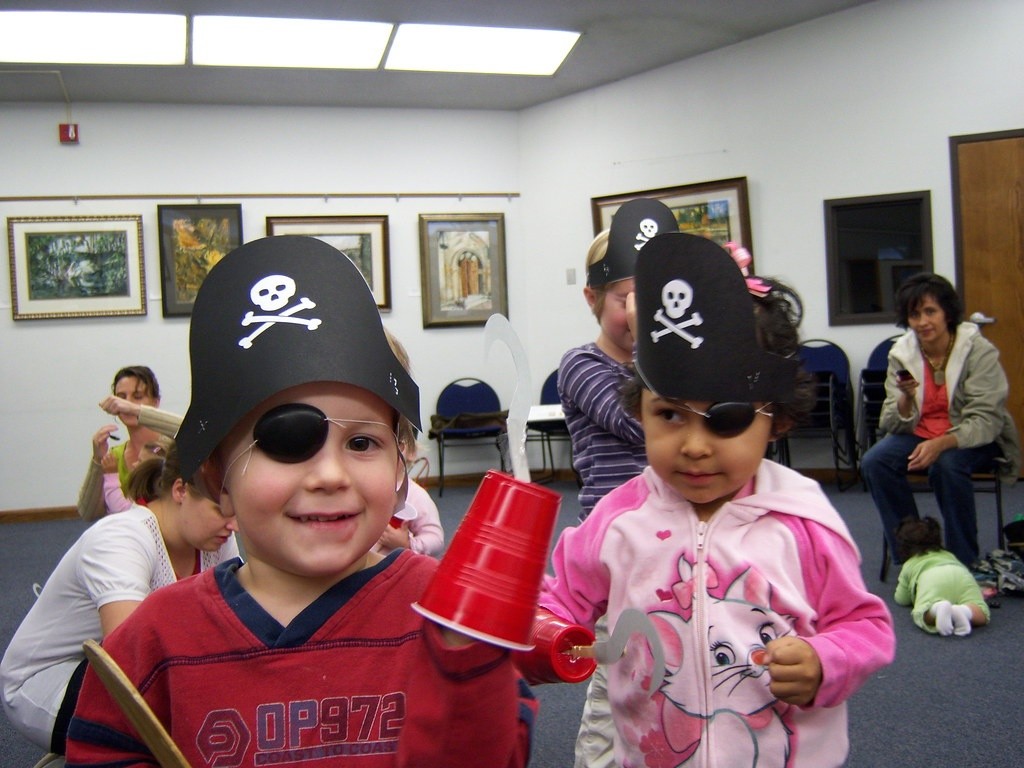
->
[265,212,394,315]
[156,202,245,317]
[588,176,757,282]
[418,210,512,332]
[6,213,150,323]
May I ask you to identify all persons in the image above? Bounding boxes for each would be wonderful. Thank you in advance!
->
[377,327,446,556]
[890,515,991,638]
[859,269,1024,571]
[511,232,896,767]
[0,437,239,768]
[557,197,681,526]
[64,235,541,768]
[77,365,185,524]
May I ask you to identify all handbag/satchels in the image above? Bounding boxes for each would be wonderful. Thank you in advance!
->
[968,549,1024,594]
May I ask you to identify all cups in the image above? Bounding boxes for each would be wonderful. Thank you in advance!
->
[410,469,563,652]
[389,502,418,531]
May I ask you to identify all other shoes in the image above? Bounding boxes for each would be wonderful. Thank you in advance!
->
[980,584,998,600]
[33,753,65,768]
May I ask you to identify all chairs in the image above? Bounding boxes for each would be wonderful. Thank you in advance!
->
[858,335,904,495]
[774,338,854,493]
[428,377,503,499]
[878,470,1006,584]
[524,367,584,487]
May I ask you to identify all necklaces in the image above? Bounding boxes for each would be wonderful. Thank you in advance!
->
[919,334,955,384]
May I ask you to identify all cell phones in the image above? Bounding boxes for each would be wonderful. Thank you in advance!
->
[896,369,916,384]
